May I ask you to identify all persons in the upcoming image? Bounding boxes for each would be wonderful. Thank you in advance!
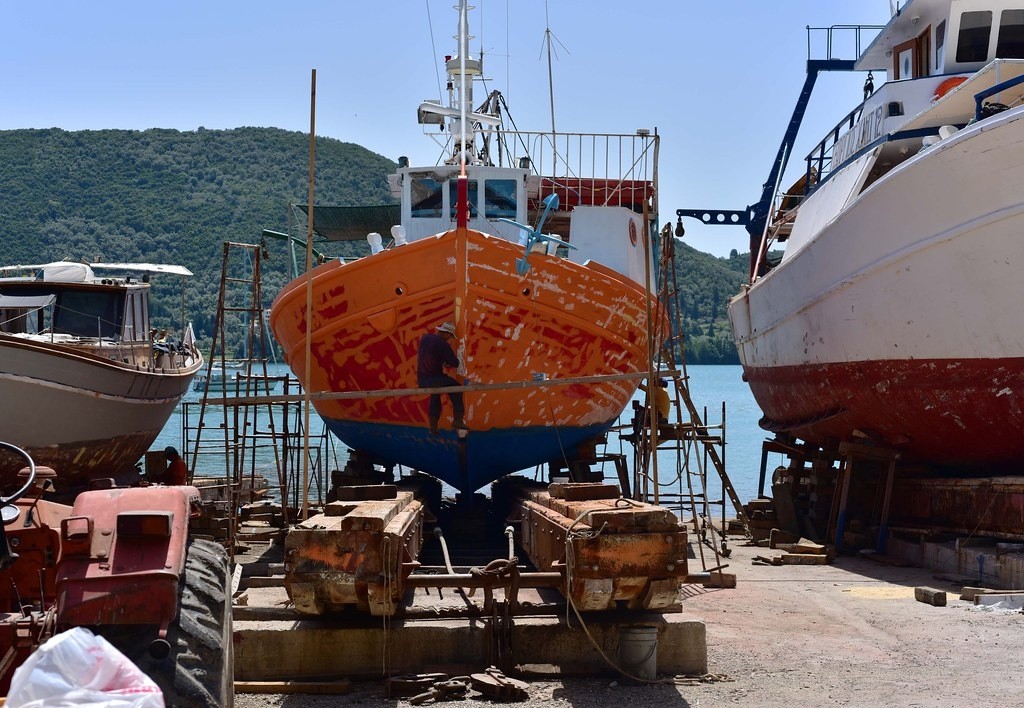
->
[417,322,471,435]
[149,327,168,366]
[626,377,670,442]
[161,446,189,486]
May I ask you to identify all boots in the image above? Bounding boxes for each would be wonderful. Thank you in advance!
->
[452,412,470,430]
[429,416,440,435]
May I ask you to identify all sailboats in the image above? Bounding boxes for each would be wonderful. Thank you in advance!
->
[192,244,281,392]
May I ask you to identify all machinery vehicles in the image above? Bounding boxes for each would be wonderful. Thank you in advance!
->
[1,443,237,708]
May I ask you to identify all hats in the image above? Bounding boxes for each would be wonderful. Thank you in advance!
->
[436,322,457,339]
[162,446,176,458]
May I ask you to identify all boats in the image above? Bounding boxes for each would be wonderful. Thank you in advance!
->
[653,362,674,382]
[1,257,204,487]
[268,0,673,487]
[676,0,1024,475]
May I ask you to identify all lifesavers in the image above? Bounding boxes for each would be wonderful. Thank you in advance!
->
[931,77,969,102]
